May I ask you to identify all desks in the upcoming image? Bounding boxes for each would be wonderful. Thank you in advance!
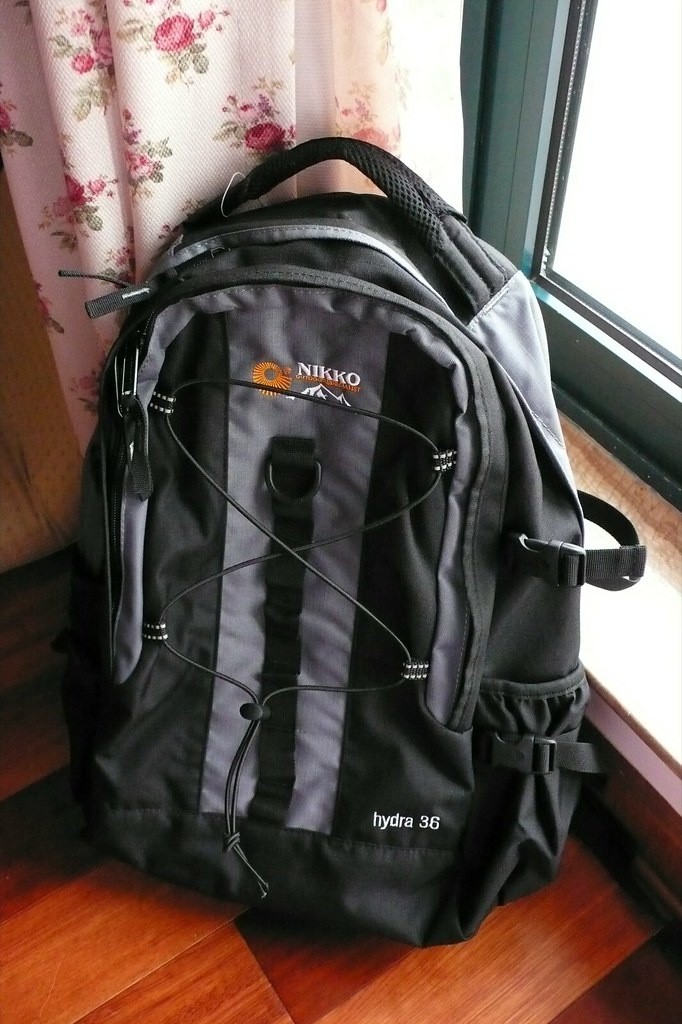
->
[0,543,663,1024]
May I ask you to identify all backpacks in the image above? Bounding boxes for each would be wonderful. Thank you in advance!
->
[52,137,645,948]
